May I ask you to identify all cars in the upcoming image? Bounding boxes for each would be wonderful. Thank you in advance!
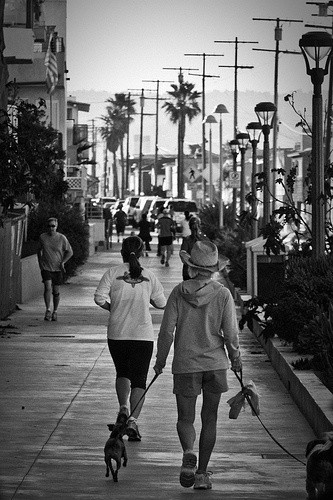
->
[159,198,200,235]
[92,195,169,223]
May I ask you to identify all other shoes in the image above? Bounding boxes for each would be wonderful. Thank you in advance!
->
[165,262,169,267]
[44,310,52,321]
[51,311,57,321]
[125,418,141,442]
[117,407,129,418]
[160,255,165,264]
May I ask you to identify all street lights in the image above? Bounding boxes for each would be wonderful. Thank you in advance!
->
[254,102,278,236]
[246,122,263,239]
[299,30,333,260]
[237,131,251,224]
[229,139,241,214]
[214,104,229,231]
[202,115,219,208]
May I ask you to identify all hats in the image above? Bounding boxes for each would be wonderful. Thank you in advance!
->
[178,239,229,273]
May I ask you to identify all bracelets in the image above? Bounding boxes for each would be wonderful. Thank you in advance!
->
[228,352,242,362]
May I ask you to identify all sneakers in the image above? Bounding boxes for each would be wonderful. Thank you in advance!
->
[179,450,198,488]
[192,470,213,490]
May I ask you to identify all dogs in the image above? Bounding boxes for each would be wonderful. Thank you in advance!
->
[305,439,333,500]
[103,422,128,482]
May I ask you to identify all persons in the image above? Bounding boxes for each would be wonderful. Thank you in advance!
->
[103,201,194,267]
[94,236,167,441]
[153,240,244,490]
[36,218,73,321]
[180,216,210,281]
